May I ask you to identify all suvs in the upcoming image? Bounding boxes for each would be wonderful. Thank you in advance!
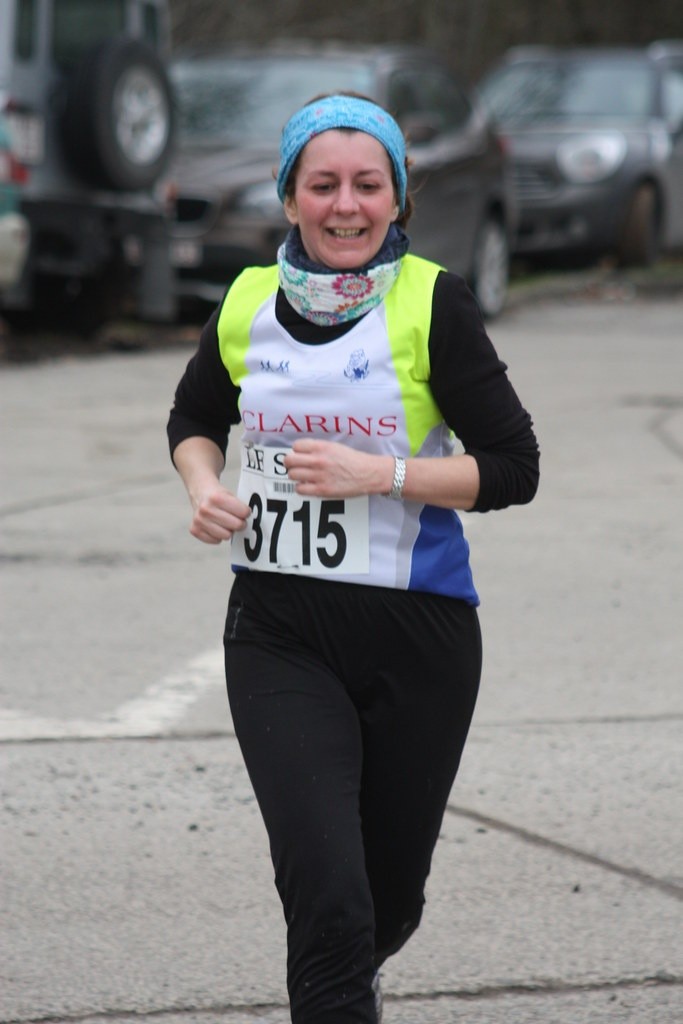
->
[156,45,516,325]
[0,0,179,337]
[478,44,683,277]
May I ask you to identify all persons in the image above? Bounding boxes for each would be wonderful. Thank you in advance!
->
[165,90,542,1023]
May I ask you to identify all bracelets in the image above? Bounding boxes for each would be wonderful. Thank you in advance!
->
[383,456,406,500]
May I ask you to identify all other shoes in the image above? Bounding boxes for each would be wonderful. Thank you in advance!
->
[371,969,383,1024]
